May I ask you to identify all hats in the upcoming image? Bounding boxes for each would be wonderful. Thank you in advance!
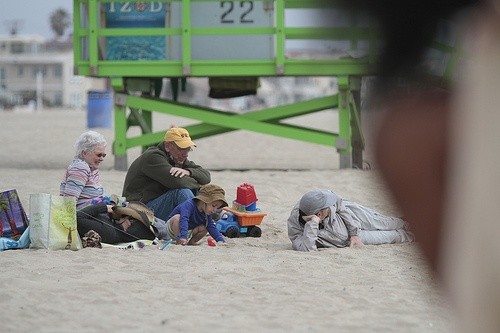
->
[192,183,229,209]
[299,189,338,216]
[164,127,198,149]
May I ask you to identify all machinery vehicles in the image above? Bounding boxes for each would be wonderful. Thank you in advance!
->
[216,207,268,238]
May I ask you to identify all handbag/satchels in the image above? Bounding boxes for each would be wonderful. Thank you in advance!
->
[28,192,83,250]
[0,189,29,237]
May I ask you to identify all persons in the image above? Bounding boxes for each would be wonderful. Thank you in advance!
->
[165,184,229,246]
[60,131,166,245]
[122,128,210,222]
[288,189,419,253]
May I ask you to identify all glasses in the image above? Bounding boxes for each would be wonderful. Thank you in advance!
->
[172,142,194,154]
[95,153,107,158]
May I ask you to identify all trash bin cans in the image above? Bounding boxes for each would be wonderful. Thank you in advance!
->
[86,89,114,129]
